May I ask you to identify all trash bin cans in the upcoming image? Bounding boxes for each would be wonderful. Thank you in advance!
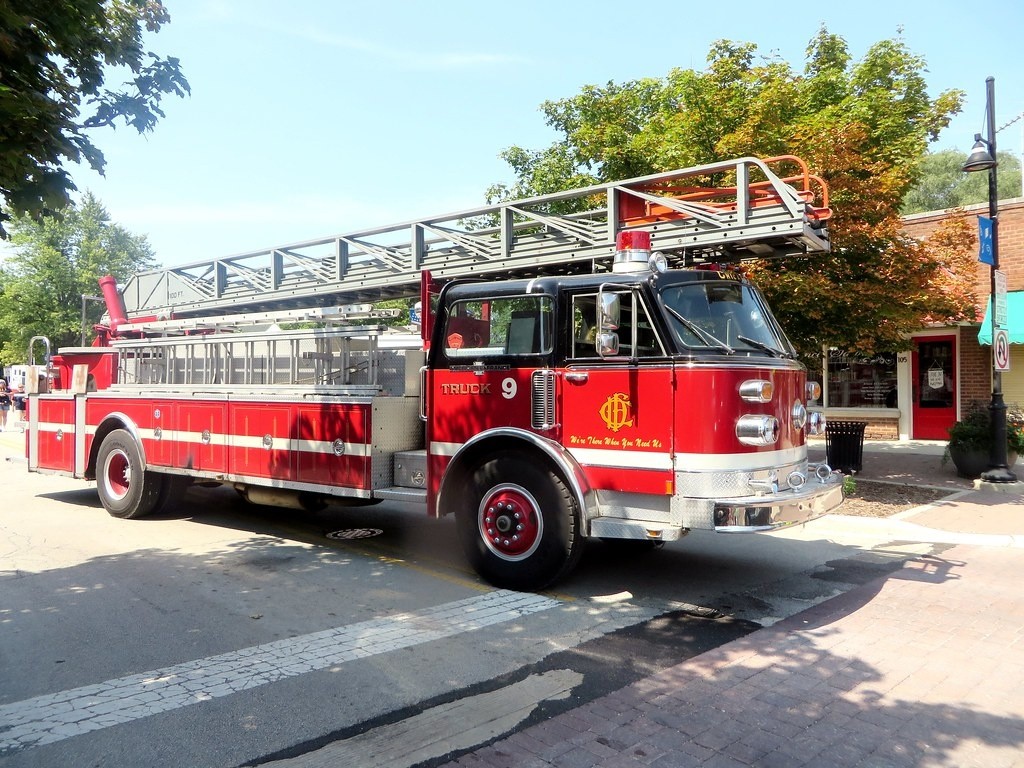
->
[824,420,869,474]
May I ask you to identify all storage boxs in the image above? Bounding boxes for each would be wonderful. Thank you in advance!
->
[391,450,428,488]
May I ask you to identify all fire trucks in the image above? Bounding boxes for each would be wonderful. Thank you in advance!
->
[6,152,846,594]
[806,344,954,409]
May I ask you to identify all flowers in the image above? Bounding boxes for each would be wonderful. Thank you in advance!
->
[1007,411,1024,457]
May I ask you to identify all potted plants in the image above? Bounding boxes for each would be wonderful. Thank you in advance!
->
[936,419,993,481]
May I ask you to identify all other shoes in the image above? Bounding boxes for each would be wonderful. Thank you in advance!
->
[0,428,3,432]
[3,428,7,432]
[20,429,24,433]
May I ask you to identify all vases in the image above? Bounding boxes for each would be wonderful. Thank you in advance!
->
[1006,450,1018,467]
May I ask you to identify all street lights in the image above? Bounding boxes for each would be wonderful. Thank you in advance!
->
[958,135,1020,483]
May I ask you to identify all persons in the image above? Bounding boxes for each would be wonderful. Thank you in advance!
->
[0,379,30,434]
